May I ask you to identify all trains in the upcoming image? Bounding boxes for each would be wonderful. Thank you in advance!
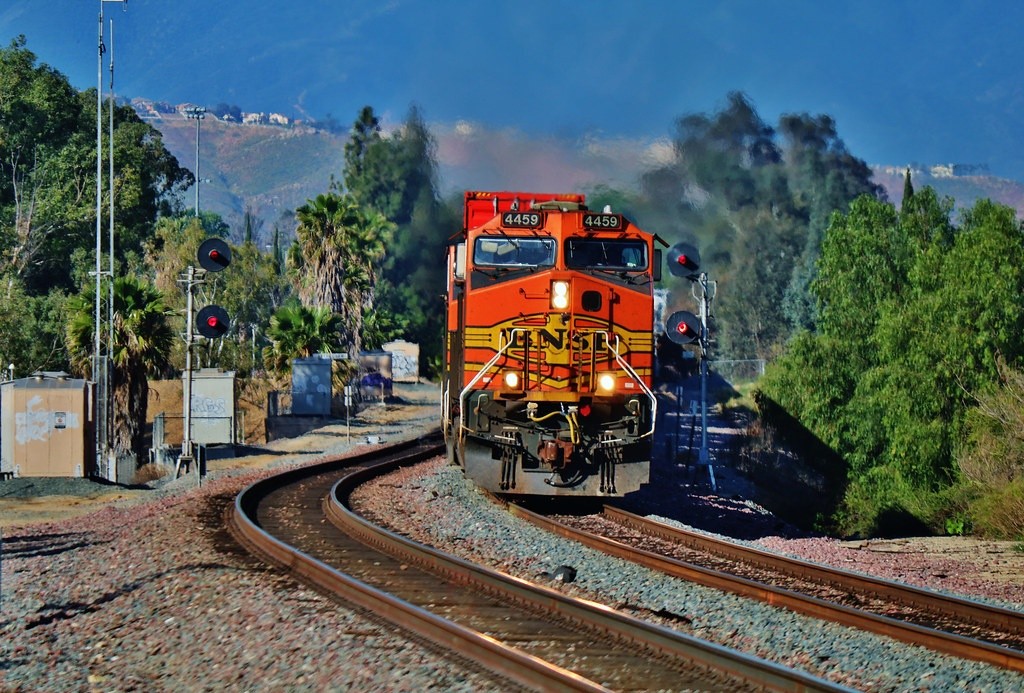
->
[438,191,671,502]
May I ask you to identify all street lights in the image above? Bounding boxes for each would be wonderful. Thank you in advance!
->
[181,105,208,217]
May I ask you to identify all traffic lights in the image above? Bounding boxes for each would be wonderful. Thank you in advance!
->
[196,305,230,338]
[667,242,700,278]
[197,238,232,272]
[665,310,700,345]
